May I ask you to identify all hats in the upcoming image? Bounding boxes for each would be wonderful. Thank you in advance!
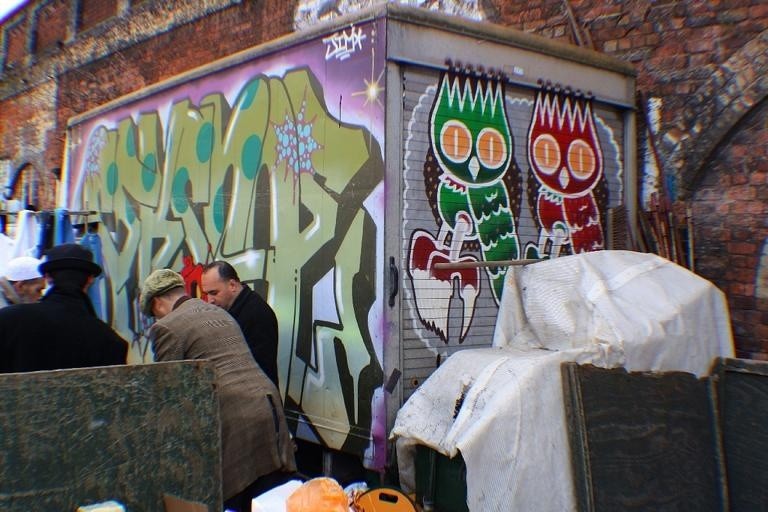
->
[2,257,43,282]
[38,244,102,277]
[139,269,185,317]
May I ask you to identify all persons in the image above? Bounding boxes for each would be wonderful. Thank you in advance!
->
[0,243,128,374]
[200,261,279,390]
[0,257,48,310]
[140,269,298,512]
